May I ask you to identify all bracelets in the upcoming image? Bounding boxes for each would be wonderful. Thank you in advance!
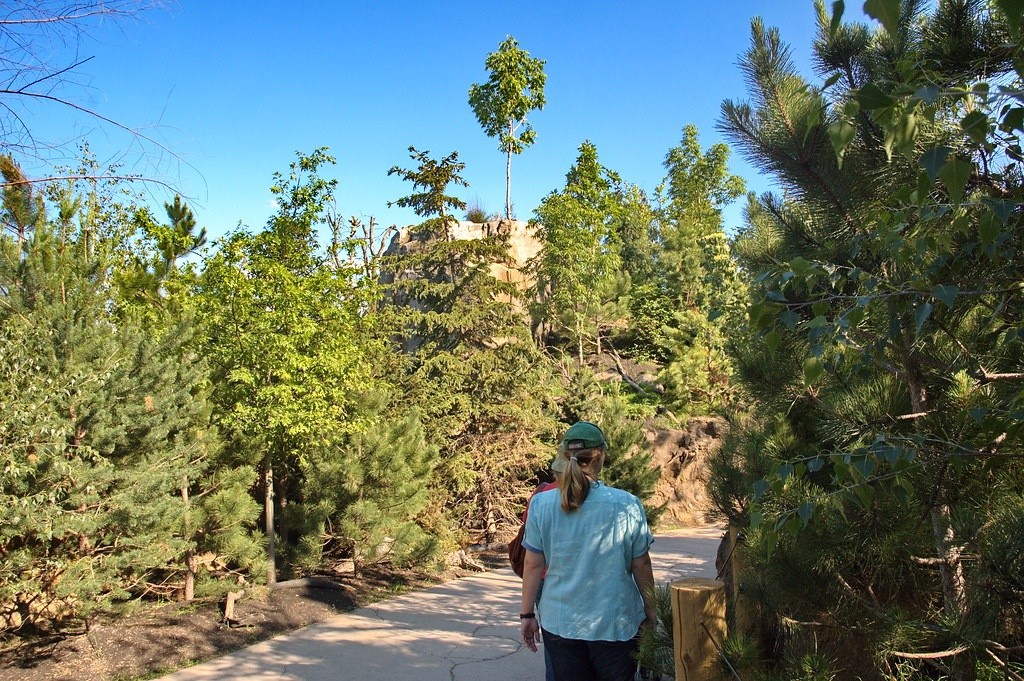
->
[520,612,535,620]
[646,601,656,609]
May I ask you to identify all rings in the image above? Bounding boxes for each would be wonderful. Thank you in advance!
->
[525,638,526,640]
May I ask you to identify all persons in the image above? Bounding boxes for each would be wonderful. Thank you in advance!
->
[521,420,657,681]
[522,441,567,681]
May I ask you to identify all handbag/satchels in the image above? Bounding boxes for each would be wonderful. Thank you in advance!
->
[508,482,548,579]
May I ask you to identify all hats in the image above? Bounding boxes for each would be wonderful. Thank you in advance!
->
[563,420,609,450]
[551,457,567,472]
